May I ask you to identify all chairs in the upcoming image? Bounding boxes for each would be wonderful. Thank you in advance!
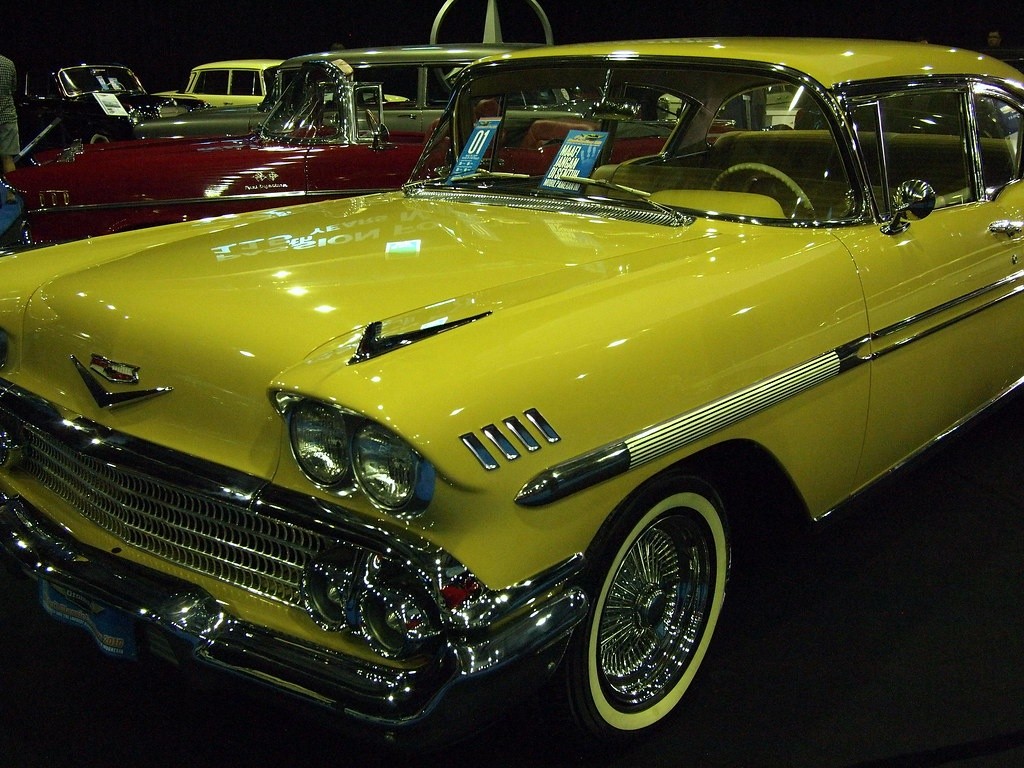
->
[424,117,507,149]
[521,120,598,149]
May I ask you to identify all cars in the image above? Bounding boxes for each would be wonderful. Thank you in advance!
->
[0,36,1023,738]
[1,45,734,265]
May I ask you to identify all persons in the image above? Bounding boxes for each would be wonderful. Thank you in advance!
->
[0,54,20,204]
[917,37,930,44]
[328,40,347,51]
[982,30,1015,67]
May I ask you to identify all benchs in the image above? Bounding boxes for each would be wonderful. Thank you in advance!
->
[703,130,1015,195]
[584,164,895,221]
